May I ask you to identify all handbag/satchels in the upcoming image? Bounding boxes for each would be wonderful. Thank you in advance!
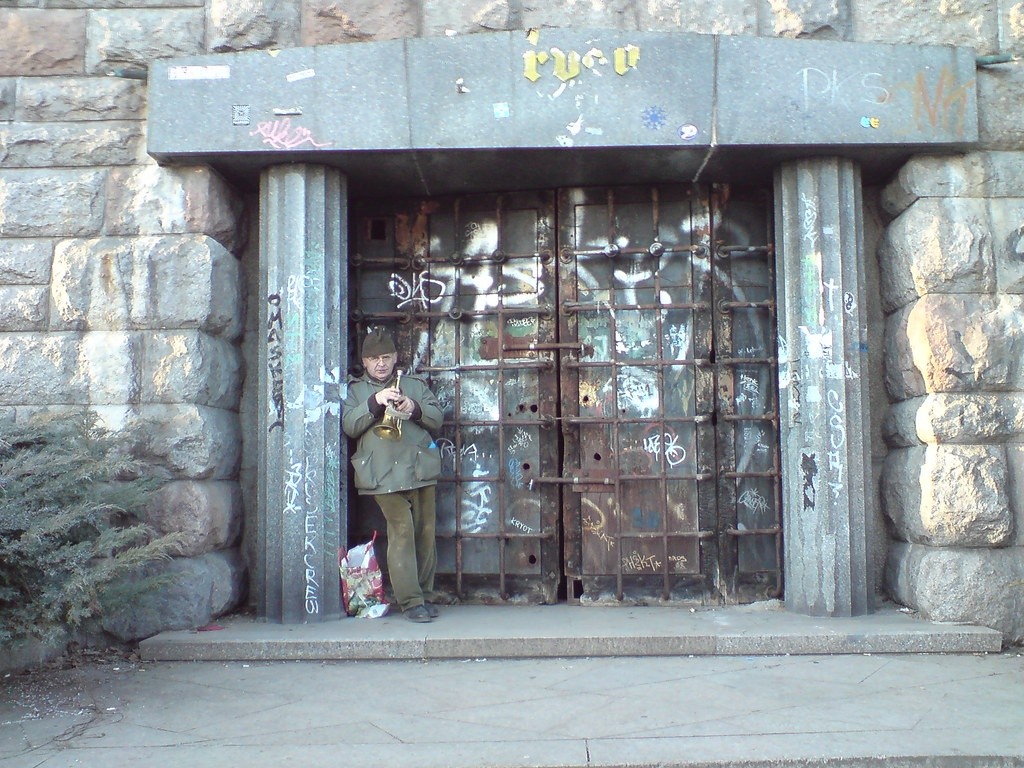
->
[338,529,390,619]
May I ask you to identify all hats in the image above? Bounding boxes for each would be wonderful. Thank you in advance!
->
[361,323,396,357]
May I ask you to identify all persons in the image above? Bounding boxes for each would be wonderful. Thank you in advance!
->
[342,330,444,623]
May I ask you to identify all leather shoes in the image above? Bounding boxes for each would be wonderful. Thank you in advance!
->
[424,602,438,617]
[402,604,431,622]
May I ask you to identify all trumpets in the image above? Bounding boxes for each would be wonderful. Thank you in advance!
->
[372,369,404,442]
